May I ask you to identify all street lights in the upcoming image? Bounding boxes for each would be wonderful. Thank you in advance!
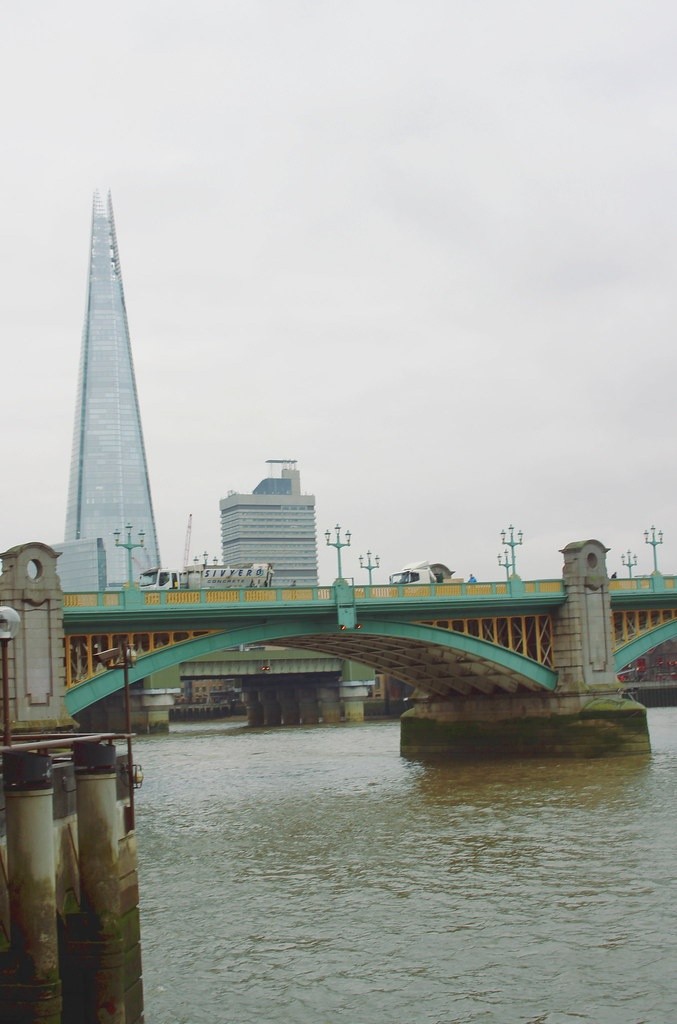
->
[324,524,352,582]
[501,524,523,575]
[497,548,516,580]
[621,549,638,577]
[193,551,218,565]
[644,525,664,571]
[0,606,21,746]
[113,523,145,590]
[358,550,380,585]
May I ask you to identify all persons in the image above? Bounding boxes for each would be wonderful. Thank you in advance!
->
[611,572,617,579]
[437,573,444,583]
[468,574,477,582]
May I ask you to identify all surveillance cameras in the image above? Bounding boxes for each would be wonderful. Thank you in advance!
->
[92,648,119,663]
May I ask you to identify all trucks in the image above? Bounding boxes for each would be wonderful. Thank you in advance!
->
[139,562,274,590]
[389,560,456,584]
